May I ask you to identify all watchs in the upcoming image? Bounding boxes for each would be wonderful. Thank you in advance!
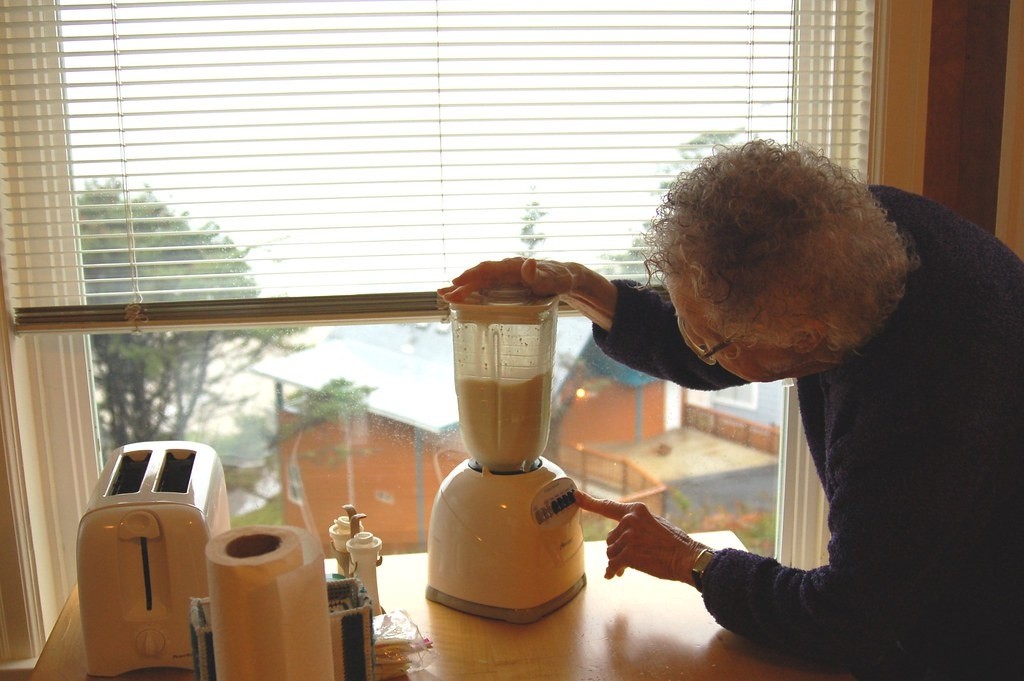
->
[691,545,718,592]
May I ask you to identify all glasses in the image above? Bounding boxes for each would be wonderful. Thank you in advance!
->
[676,314,755,366]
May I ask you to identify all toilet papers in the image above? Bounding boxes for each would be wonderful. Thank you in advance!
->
[202,524,336,681]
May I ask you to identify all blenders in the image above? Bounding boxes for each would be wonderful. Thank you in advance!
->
[425,287,587,624]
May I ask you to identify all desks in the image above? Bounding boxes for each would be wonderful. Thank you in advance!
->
[31,530,847,681]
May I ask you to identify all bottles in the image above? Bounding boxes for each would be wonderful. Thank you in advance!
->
[346,531,383,616]
[329,516,364,577]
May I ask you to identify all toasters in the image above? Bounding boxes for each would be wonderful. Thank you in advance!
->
[74,440,231,678]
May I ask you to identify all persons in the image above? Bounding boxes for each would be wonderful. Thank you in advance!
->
[437,140,1024,681]
